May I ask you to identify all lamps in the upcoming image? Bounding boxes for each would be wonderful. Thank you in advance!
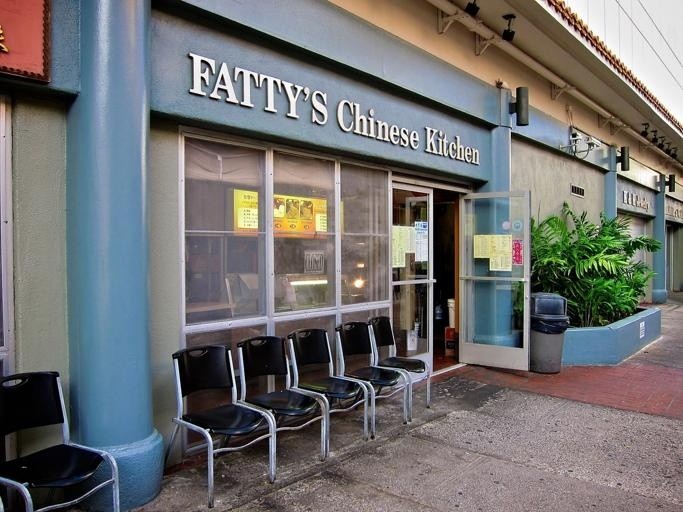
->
[616,146,629,171]
[509,87,529,126]
[502,14,516,41]
[664,175,675,192]
[640,122,678,159]
[464,0,480,17]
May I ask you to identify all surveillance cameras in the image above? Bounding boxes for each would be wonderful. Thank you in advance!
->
[568,131,582,145]
[583,136,601,151]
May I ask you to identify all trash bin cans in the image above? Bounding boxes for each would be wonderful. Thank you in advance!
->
[530,292,569,373]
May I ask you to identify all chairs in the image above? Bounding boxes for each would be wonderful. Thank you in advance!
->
[0,370,120,512]
